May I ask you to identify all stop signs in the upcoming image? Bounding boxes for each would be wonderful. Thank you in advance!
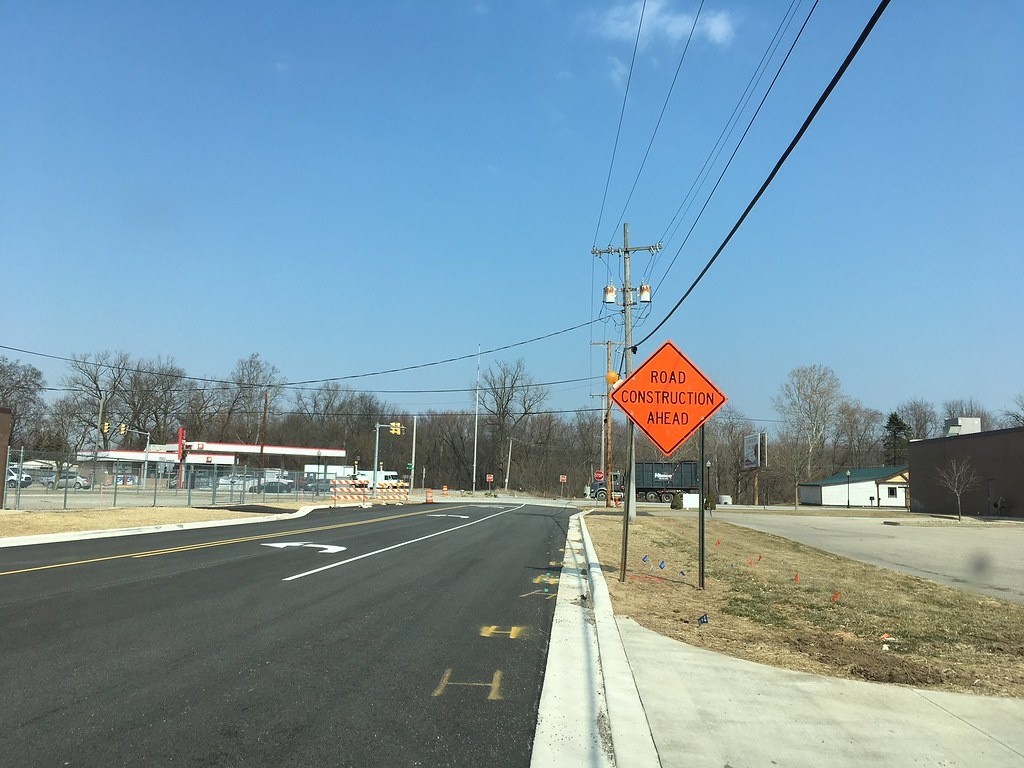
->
[594,470,604,481]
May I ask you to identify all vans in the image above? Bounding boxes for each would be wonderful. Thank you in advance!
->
[6,467,33,489]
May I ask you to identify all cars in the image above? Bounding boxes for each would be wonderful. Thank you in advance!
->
[39,472,91,490]
[250,482,291,493]
[288,476,335,493]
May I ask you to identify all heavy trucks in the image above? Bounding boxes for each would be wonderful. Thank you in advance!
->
[589,459,699,504]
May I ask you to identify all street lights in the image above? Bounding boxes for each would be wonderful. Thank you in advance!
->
[846,470,851,508]
[315,449,322,495]
[706,460,711,495]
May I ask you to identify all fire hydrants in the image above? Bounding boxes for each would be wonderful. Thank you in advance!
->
[614,497,621,507]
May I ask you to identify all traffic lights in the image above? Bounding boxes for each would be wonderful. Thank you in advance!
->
[104,423,110,433]
[121,422,127,435]
[395,422,401,436]
[389,422,395,434]
